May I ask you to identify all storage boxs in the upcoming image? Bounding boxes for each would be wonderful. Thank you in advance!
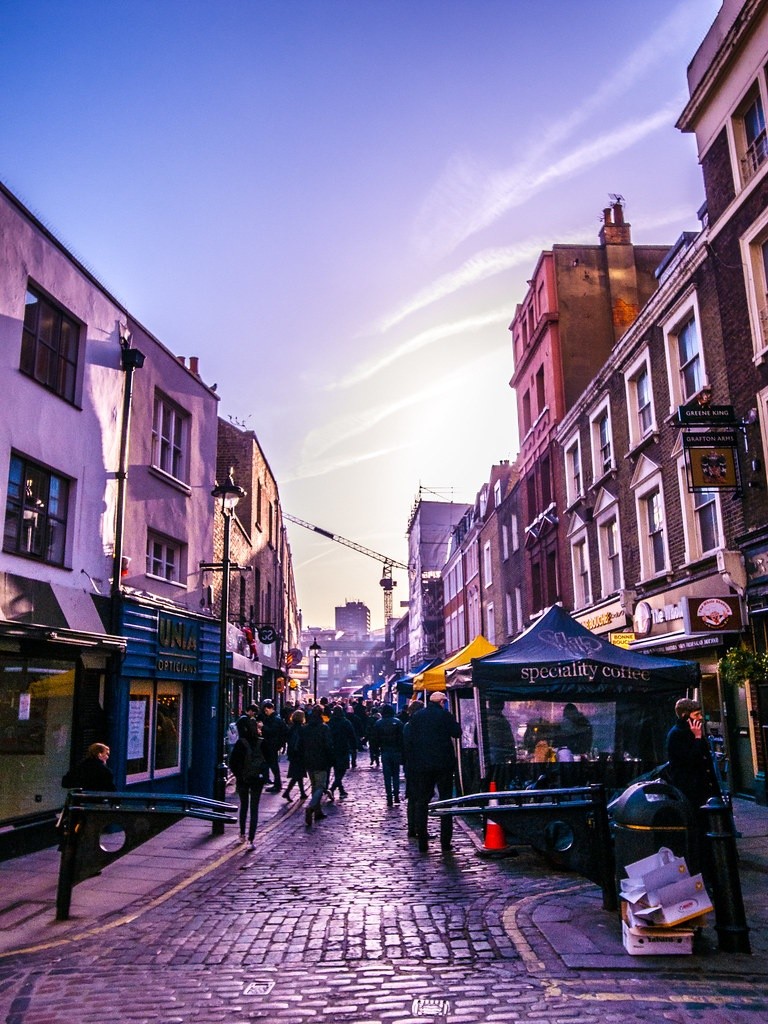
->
[621,902,709,955]
[626,901,710,931]
[619,847,690,909]
[634,873,714,928]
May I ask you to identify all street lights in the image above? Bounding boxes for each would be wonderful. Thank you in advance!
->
[309,635,321,703]
[210,467,247,837]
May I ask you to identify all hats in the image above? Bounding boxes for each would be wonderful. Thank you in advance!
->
[429,691,447,701]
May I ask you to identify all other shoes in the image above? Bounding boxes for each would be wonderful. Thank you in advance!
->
[327,789,335,801]
[441,843,453,852]
[339,793,349,799]
[306,806,313,826]
[313,810,328,820]
[265,786,282,793]
[426,833,437,840]
[300,795,308,799]
[246,842,256,851]
[418,844,428,852]
[387,801,394,806]
[394,797,400,803]
[239,834,246,843]
[407,831,419,839]
[89,871,102,878]
[282,793,294,803]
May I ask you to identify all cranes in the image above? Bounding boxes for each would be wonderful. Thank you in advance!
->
[281,511,407,626]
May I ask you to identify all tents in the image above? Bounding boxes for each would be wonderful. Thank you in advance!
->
[356,604,704,804]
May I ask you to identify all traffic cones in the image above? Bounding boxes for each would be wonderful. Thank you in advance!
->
[478,780,511,851]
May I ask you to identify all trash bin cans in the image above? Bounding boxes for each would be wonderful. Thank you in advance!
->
[613,781,691,930]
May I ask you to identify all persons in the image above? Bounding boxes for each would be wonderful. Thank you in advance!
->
[229,717,268,851]
[241,697,409,823]
[562,703,593,755]
[68,744,115,881]
[402,691,462,851]
[660,697,712,852]
[473,699,517,763]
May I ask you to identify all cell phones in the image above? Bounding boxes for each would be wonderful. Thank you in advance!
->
[683,714,694,723]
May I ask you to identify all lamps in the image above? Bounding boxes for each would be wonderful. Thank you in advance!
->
[574,258,579,267]
[729,490,742,501]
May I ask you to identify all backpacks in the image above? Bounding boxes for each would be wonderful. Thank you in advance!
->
[241,736,270,783]
[227,714,247,744]
